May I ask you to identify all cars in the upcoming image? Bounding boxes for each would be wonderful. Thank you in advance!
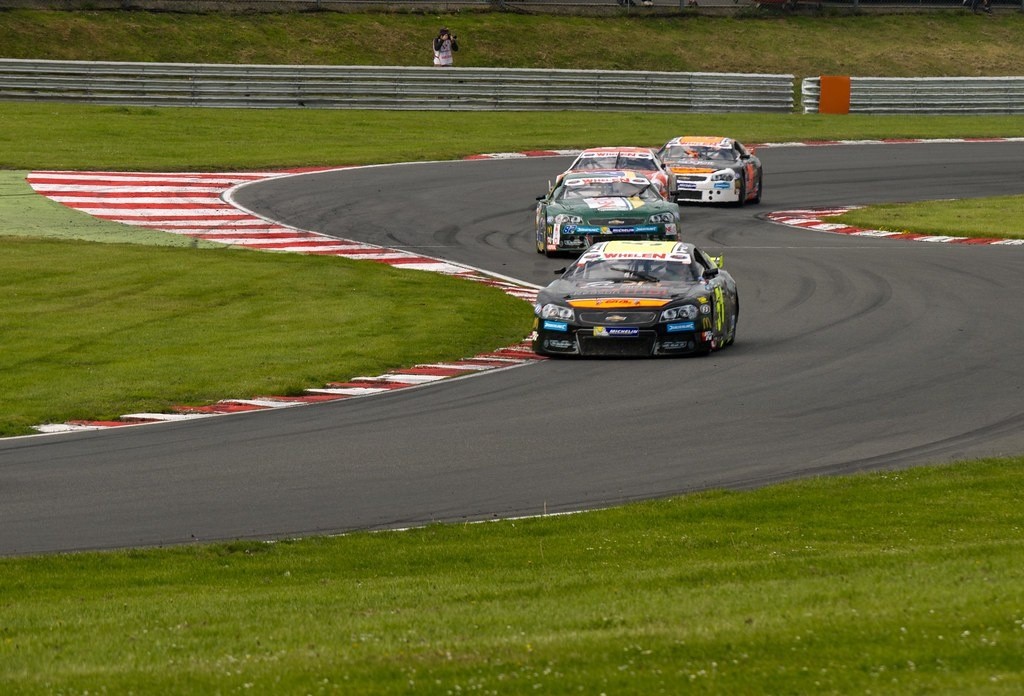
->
[530,240,741,358]
[657,136,762,208]
[556,146,677,203]
[534,172,681,259]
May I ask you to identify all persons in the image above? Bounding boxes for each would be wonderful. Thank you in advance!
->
[432,28,459,68]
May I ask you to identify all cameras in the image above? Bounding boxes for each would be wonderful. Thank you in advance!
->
[447,33,457,40]
